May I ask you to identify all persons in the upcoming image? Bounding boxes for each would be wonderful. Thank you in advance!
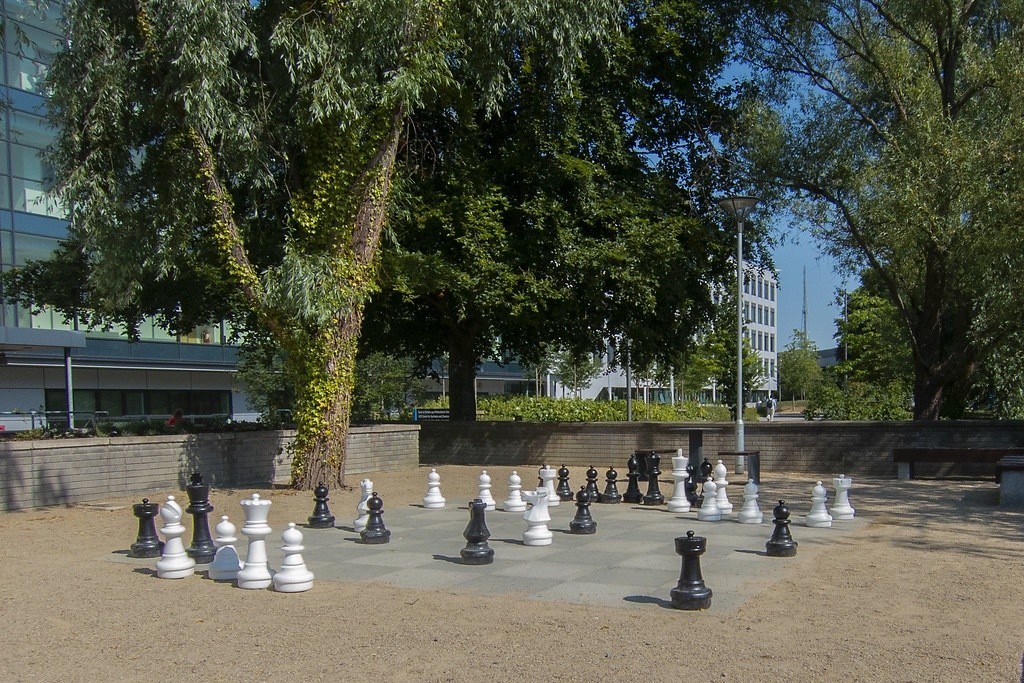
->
[169,407,183,426]
[766,394,776,421]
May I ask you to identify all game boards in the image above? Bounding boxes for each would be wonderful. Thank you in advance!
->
[97,484,896,618]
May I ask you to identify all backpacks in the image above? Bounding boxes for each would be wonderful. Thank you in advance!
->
[767,400,772,407]
